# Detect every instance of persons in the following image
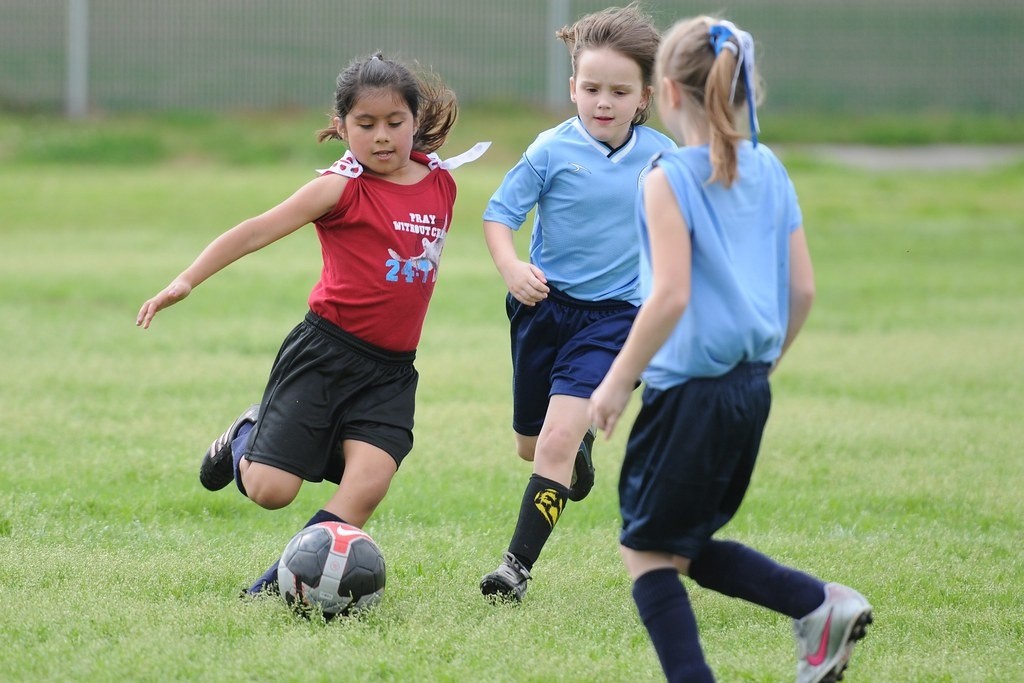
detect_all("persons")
[136,51,457,594]
[587,16,874,683]
[480,6,680,606]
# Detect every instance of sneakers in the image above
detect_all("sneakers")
[569,426,597,502]
[479,550,534,608]
[200,403,262,491]
[791,577,874,682]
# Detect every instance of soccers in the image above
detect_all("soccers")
[276,521,389,626]
